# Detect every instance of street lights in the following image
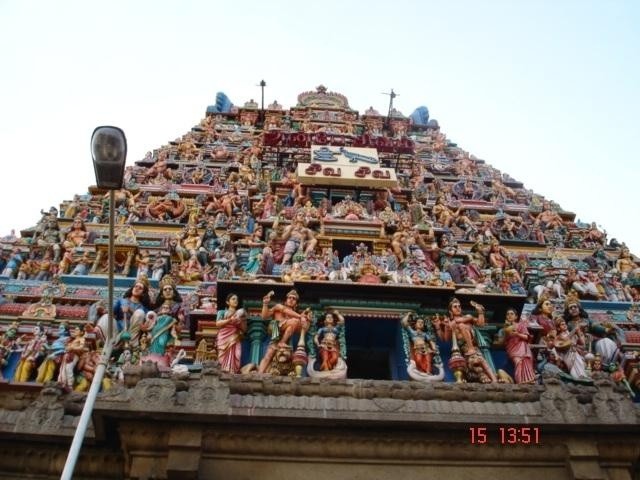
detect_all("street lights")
[395,142,404,174]
[58,127,126,480]
[275,139,282,167]
[255,80,266,130]
[384,89,396,129]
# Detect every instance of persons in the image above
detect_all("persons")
[2,110,640,393]
